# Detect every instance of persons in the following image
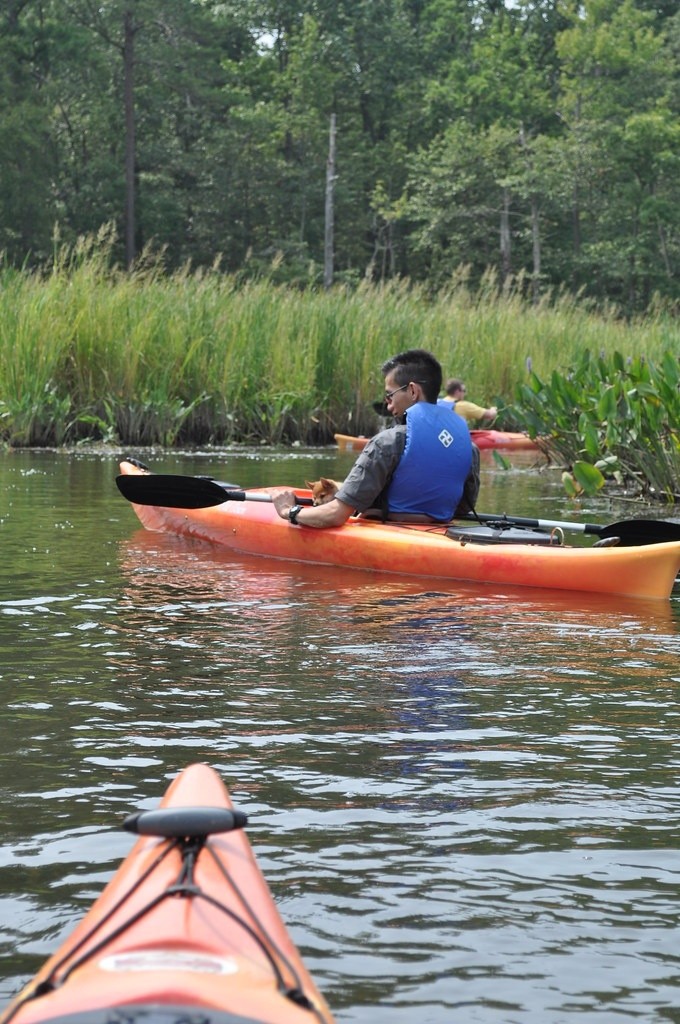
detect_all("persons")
[271,349,482,531]
[437,378,498,424]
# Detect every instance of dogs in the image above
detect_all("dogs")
[304,476,341,507]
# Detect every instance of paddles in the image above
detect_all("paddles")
[114,473,680,548]
[372,402,394,418]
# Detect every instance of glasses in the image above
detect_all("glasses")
[385,380,427,402]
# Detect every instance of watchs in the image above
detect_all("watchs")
[288,505,304,525]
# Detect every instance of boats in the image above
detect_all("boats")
[120,455,680,602]
[334,429,560,457]
